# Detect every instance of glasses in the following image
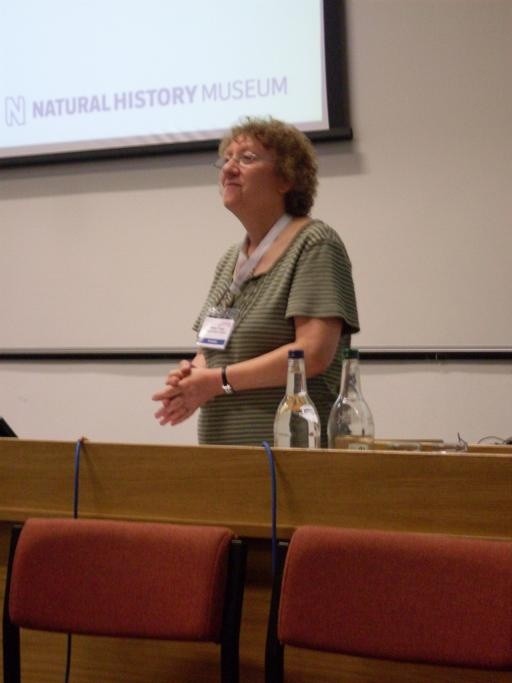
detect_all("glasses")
[215,149,278,168]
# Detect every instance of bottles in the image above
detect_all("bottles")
[272,347,323,448]
[324,348,378,448]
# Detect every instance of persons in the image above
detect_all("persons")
[151,113,361,449]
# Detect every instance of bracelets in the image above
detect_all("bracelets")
[221,363,239,395]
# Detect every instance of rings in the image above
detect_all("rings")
[183,406,189,412]
